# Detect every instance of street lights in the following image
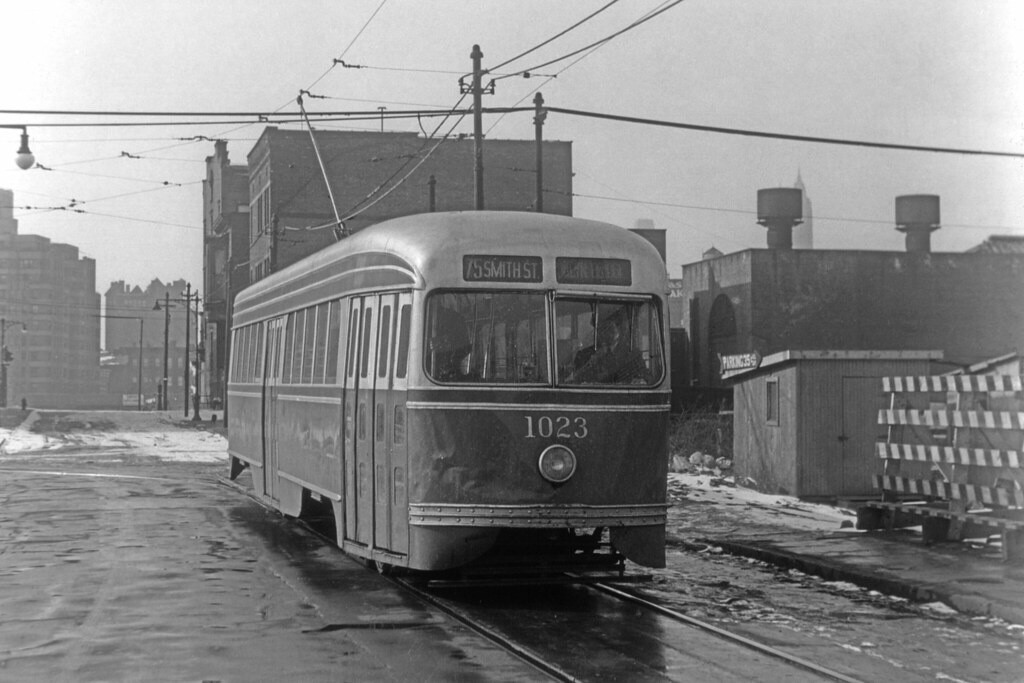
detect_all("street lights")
[0,319,28,410]
[152,298,201,421]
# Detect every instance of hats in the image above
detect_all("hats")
[590,308,624,326]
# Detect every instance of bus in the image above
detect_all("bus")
[227,209,673,591]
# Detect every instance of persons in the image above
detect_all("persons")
[575,307,650,385]
[428,307,471,380]
